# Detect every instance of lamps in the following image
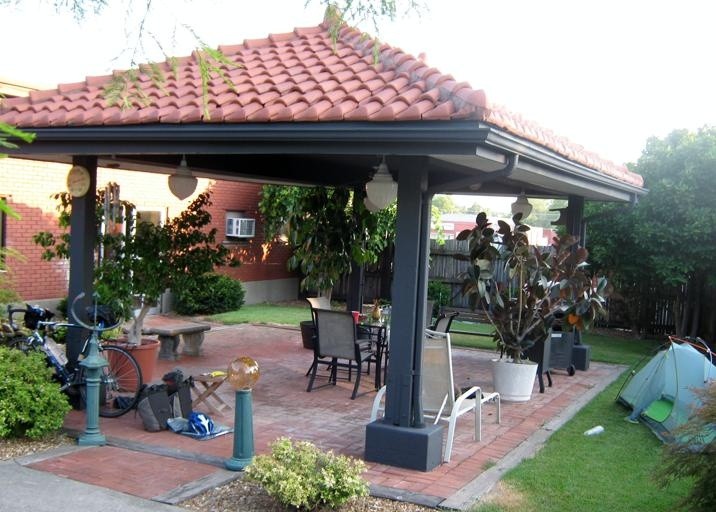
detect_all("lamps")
[167,154,198,198]
[365,154,400,211]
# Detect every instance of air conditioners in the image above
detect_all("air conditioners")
[227,218,257,240]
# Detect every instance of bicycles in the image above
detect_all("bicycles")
[7,298,143,421]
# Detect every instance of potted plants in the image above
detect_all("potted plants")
[452,210,614,402]
[257,185,447,351]
[30,185,162,386]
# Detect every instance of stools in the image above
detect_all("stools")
[174,371,230,416]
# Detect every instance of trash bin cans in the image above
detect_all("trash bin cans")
[300,321,315,349]
[548,318,576,376]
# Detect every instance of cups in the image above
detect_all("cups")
[353,311,359,324]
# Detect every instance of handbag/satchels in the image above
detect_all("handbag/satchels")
[137,391,173,431]
[170,385,193,418]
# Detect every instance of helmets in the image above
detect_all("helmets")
[188,412,214,439]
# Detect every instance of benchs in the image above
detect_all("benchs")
[142,321,207,359]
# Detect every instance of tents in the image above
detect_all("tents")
[613,333,716,459]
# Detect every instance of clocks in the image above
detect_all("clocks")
[66,167,90,200]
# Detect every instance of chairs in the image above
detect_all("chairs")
[281,297,501,464]
